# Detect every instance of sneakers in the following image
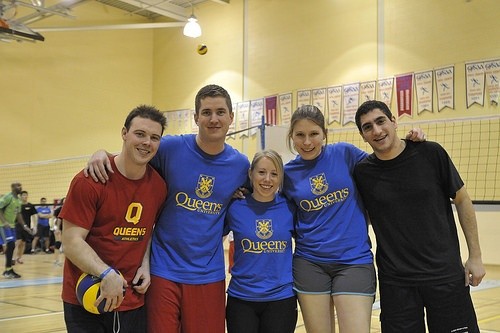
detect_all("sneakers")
[3,268,21,278]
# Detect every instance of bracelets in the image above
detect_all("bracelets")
[99,266,120,278]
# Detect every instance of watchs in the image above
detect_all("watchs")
[22,224,28,228]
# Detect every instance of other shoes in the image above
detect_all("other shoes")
[45,249,52,254]
[12,259,16,265]
[16,257,23,264]
[30,249,35,253]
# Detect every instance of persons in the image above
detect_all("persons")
[353,100,486,333]
[0,85,426,333]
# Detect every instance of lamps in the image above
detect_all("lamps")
[182,3,202,38]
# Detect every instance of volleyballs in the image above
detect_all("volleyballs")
[197,44,208,55]
[76,266,127,314]
